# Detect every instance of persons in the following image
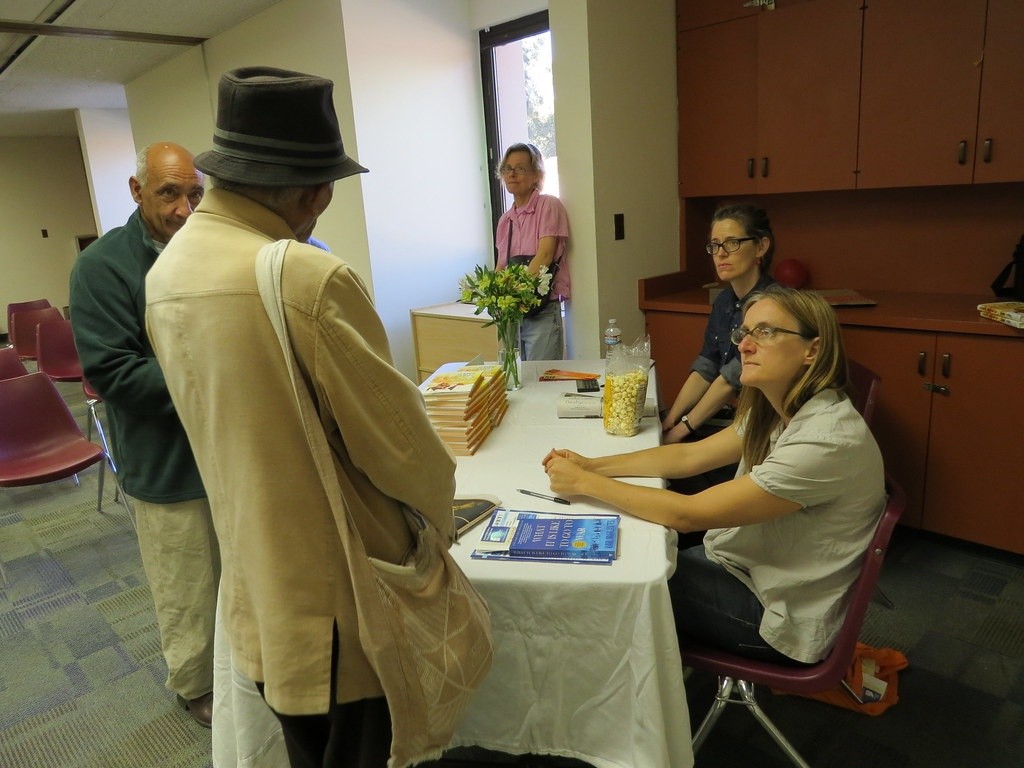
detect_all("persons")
[145,64,457,768]
[495,142,571,361]
[70,142,222,728]
[658,204,786,552]
[542,286,890,682]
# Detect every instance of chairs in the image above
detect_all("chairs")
[0,298,141,540]
[839,355,881,428]
[684,469,906,768]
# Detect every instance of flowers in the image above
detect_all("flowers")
[455,260,553,388]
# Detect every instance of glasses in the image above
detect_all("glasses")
[731,324,803,344]
[500,164,531,175]
[705,237,753,255]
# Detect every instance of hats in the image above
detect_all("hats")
[194,67,369,187]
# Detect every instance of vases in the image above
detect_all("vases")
[494,320,523,390]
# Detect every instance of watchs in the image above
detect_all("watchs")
[681,416,697,434]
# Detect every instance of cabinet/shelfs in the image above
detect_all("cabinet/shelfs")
[637,0,1024,556]
[410,300,503,384]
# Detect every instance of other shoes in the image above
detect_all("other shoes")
[176,692,212,729]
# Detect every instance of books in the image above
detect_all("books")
[415,365,510,458]
[555,390,657,419]
[540,368,601,381]
[467,508,620,565]
[976,300,1024,331]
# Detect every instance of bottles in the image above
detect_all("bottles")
[604,319,622,367]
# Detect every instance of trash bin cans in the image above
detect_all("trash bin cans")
[63,305,70,320]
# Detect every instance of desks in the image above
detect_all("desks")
[418,358,695,768]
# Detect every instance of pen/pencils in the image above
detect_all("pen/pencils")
[516,488,570,506]
[600,384,605,387]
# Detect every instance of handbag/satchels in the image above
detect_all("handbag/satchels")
[363,529,492,768]
